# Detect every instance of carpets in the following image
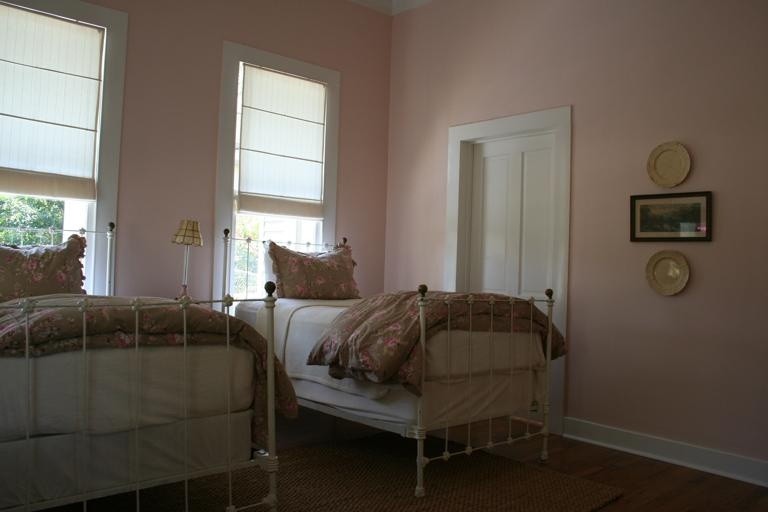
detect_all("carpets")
[29,430,626,511]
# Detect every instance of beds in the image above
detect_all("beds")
[0,221,278,511]
[222,228,554,499]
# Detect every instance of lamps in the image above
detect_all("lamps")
[170,219,204,302]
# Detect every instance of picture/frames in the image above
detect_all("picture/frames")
[629,191,713,243]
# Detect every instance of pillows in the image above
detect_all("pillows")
[267,237,362,300]
[0,233,87,304]
